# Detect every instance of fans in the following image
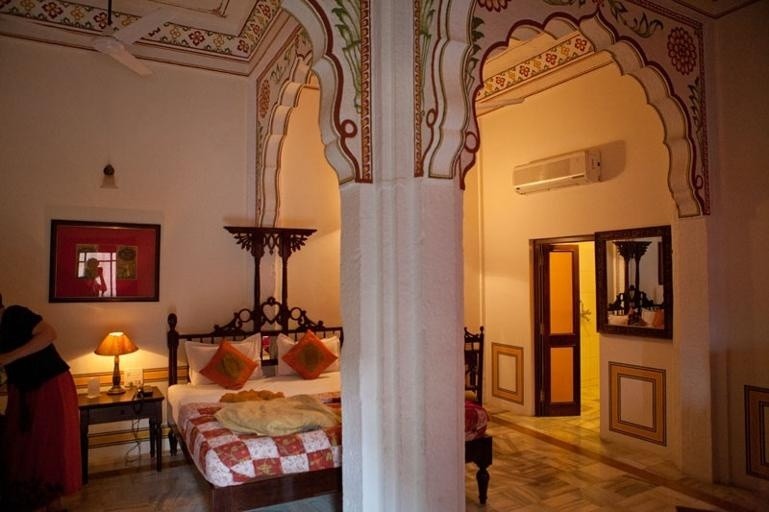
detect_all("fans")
[0,0,179,80]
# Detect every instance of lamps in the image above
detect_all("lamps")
[92,330,140,394]
[100,51,119,190]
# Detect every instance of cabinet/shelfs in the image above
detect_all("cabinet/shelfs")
[465,322,485,405]
[76,386,164,483]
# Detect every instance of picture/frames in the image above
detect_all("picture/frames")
[596,225,676,340]
[48,218,161,303]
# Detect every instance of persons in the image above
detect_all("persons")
[79,257,108,298]
[0,296,87,510]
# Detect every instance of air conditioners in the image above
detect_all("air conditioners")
[512,146,602,196]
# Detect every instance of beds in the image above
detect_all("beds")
[163,297,493,512]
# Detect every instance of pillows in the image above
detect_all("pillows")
[282,330,337,379]
[184,332,264,387]
[198,341,258,390]
[275,332,341,376]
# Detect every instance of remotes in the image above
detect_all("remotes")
[143,384,153,396]
